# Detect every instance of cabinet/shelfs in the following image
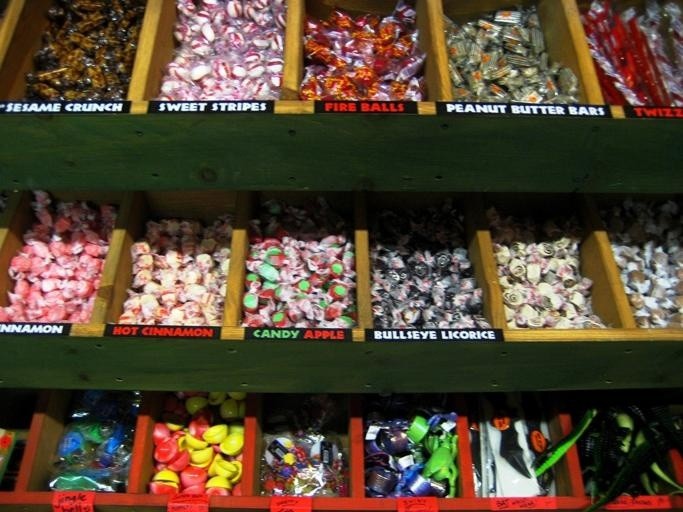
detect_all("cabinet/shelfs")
[0,188,682,341]
[0,0,683,116]
[0,384,682,511]
